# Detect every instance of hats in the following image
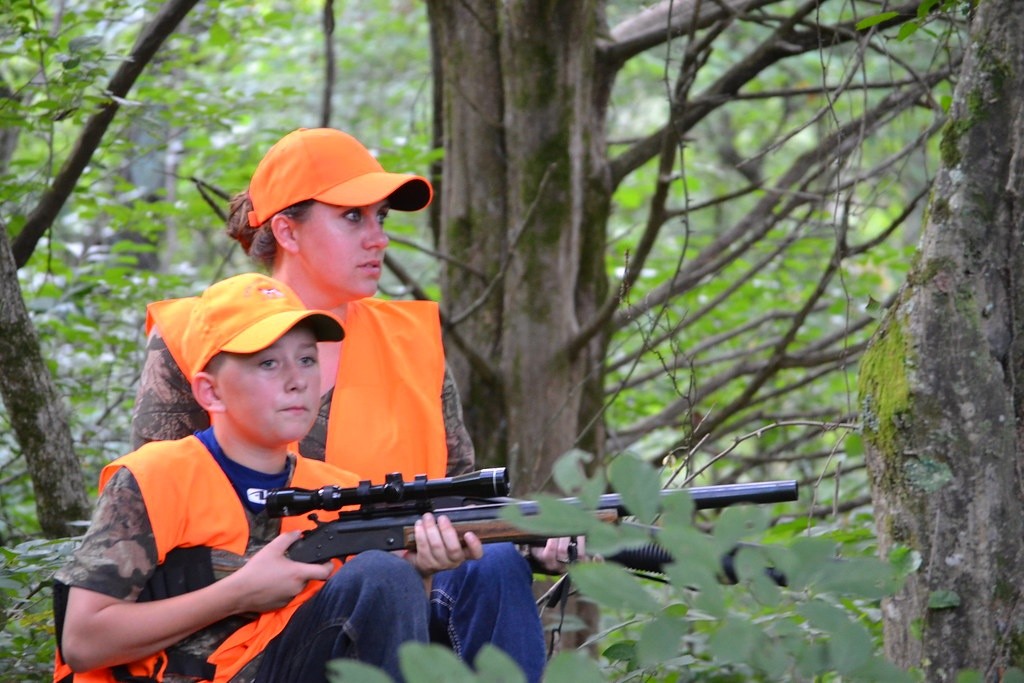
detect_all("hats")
[246,126,434,228]
[181,272,345,379]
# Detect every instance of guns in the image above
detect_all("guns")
[435,493,787,596]
[134,465,800,614]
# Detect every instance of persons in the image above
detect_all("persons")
[130,127,606,598]
[52,272,547,683]
